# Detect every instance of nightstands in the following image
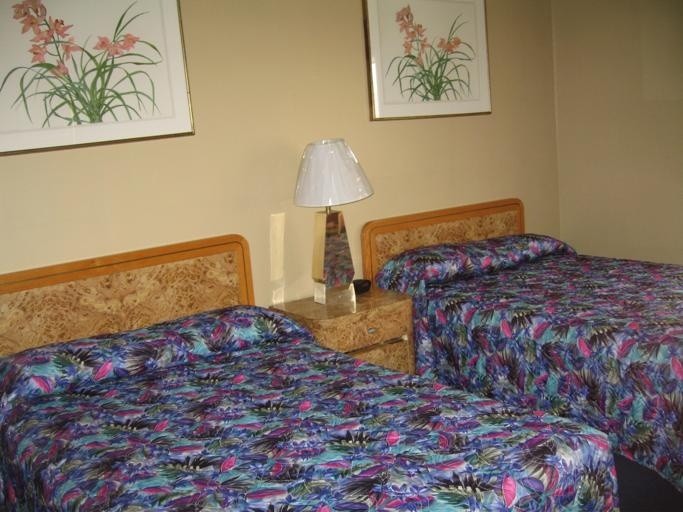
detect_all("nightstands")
[268,286,415,375]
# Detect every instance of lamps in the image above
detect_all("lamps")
[294,138,374,305]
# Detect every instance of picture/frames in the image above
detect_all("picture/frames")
[362,0,491,121]
[0,0,195,156]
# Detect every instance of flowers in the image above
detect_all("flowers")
[0,0,165,128]
[385,4,476,104]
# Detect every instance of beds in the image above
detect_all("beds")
[0,234,620,512]
[360,198,683,493]
